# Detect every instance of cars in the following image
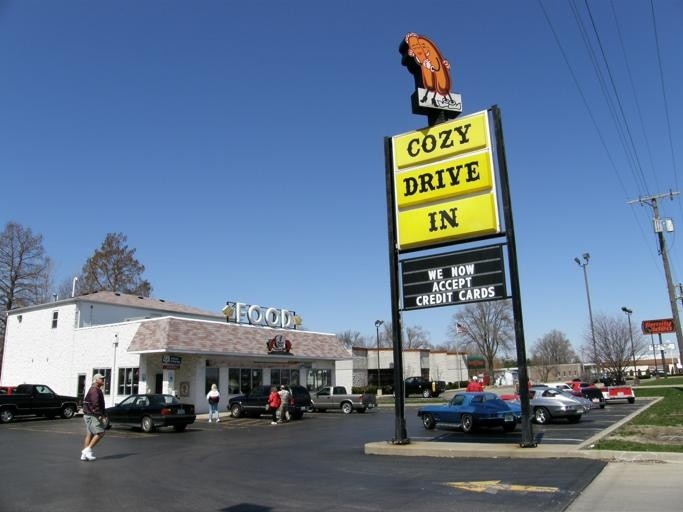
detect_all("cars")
[0,386,17,395]
[415,392,521,433]
[650,370,668,377]
[494,370,636,408]
[516,387,590,426]
[103,394,196,434]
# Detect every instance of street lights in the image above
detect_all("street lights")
[645,327,657,371]
[573,257,599,382]
[620,307,636,379]
[374,318,383,396]
[454,321,460,388]
[112,333,119,406]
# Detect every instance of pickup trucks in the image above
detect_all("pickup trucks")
[304,386,376,415]
[0,384,78,425]
[390,377,447,401]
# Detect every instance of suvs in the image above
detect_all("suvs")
[225,384,311,421]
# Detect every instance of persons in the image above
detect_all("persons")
[267,386,281,424]
[277,385,293,424]
[206,384,220,422]
[79,373,106,461]
[465,376,484,392]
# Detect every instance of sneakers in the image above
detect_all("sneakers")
[81,447,96,461]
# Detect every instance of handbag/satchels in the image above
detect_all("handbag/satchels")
[209,397,219,405]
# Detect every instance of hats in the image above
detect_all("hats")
[93,373,105,380]
